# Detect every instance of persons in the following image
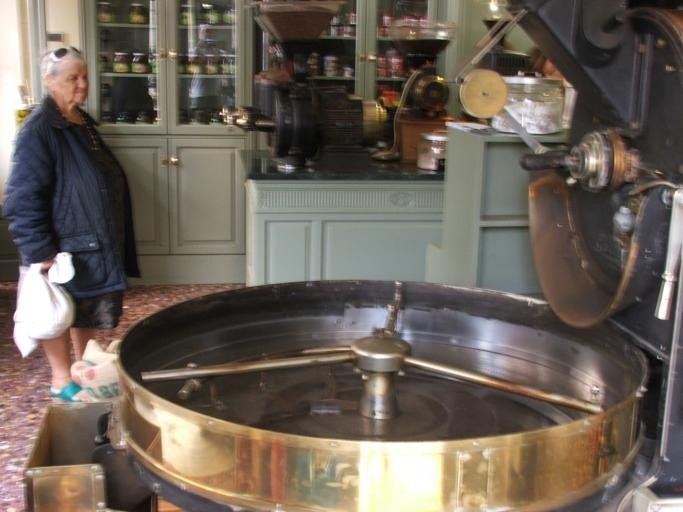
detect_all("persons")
[0,47,141,403]
[530,46,567,82]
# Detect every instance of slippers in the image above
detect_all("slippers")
[48,381,82,402]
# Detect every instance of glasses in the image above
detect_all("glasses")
[50,46,81,62]
